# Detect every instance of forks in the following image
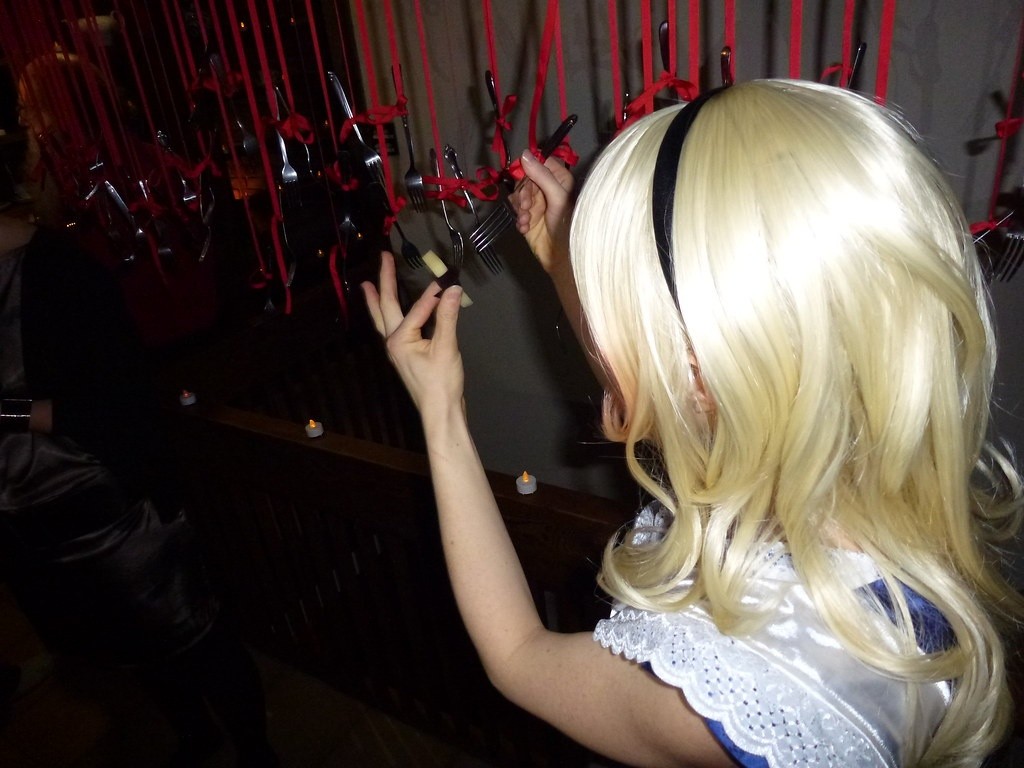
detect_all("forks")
[469,114,579,252]
[382,194,426,270]
[100,66,386,297]
[485,70,515,196]
[443,143,505,275]
[995,214,1024,284]
[429,147,464,270]
[391,63,427,214]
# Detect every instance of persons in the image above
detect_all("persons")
[0,51,282,767]
[361,77,1024,768]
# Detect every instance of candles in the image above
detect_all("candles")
[304,419,324,439]
[515,471,537,496]
[179,389,197,406]
